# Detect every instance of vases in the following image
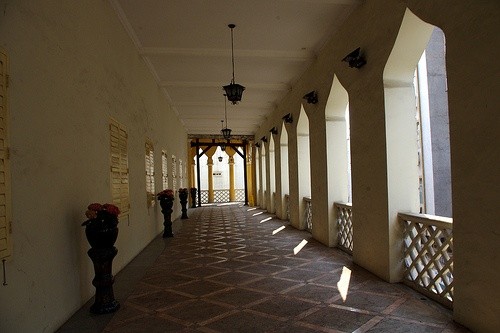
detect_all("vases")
[160,201,173,208]
[85,223,118,249]
[191,191,196,194]
[179,194,187,200]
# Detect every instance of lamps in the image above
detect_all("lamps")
[302,91,318,104]
[255,142,260,148]
[261,135,267,143]
[341,47,367,69]
[281,113,293,123]
[269,127,278,135]
[221,142,226,151]
[221,94,232,139]
[222,24,245,106]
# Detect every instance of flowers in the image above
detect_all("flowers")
[81,202,120,226]
[157,189,174,200]
[178,188,188,193]
[190,188,197,191]
